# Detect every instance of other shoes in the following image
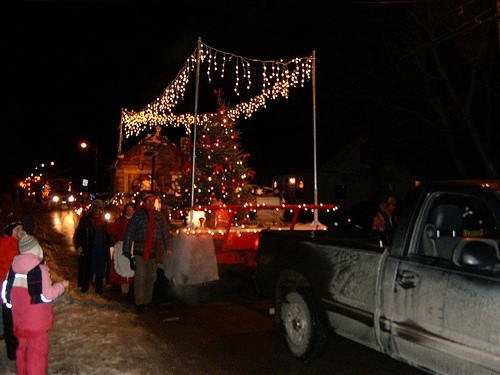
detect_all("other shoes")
[95,287,104,295]
[82,284,90,292]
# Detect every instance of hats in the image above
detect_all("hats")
[2,208,25,236]
[17,230,44,258]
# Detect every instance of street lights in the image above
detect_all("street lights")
[81,143,98,195]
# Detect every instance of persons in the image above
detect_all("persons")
[107,202,141,296]
[122,192,173,312]
[72,198,110,297]
[1,214,30,360]
[372,195,400,232]
[1,229,70,375]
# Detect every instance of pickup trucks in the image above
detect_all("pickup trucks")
[254,180,500,375]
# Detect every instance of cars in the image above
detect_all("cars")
[49,191,75,210]
